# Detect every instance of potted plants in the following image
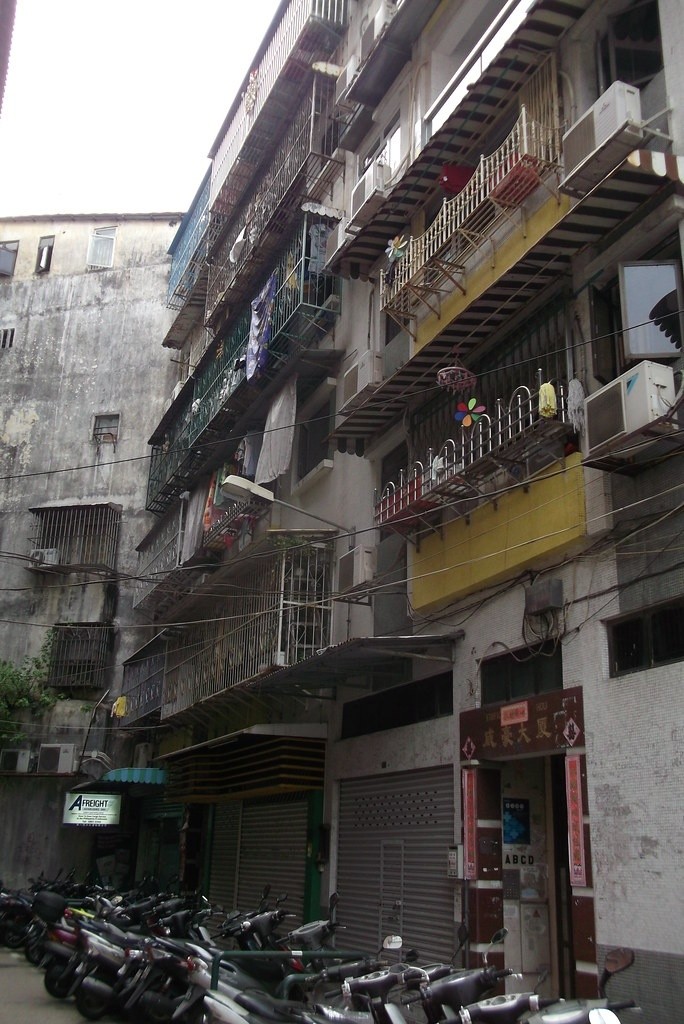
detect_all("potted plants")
[271,636,286,665]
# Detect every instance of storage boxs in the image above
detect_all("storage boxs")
[488,153,538,209]
[375,464,477,532]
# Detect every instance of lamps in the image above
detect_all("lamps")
[224,475,353,541]
[176,803,203,835]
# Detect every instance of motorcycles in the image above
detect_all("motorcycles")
[203,925,634,1024]
[0,867,346,1023]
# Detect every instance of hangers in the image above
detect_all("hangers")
[441,149,475,170]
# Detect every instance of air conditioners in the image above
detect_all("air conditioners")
[339,543,377,594]
[560,80,642,189]
[30,547,57,568]
[1,748,31,773]
[349,161,390,225]
[334,347,385,411]
[325,218,356,265]
[191,573,210,594]
[35,742,79,774]
[579,361,677,452]
[334,55,360,109]
[161,381,184,418]
[359,1,398,63]
[134,745,152,768]
